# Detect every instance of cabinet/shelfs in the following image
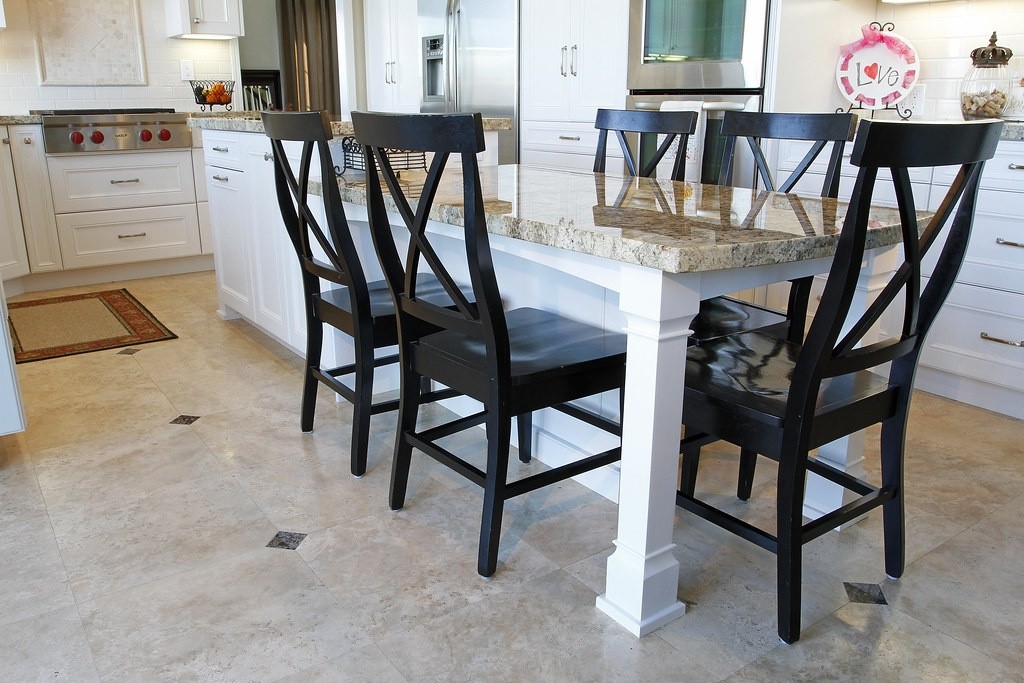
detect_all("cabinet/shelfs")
[203,127,360,375]
[361,0,420,114]
[767,130,1023,419]
[517,0,632,177]
[0,123,216,301]
[164,0,245,40]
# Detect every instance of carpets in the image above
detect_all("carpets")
[5,288,179,364]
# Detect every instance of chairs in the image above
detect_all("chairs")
[351,110,628,577]
[262,110,501,478]
[679,112,859,500]
[592,108,697,180]
[675,119,1005,647]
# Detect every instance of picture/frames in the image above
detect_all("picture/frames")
[241,69,283,110]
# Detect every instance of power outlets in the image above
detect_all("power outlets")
[180,59,195,80]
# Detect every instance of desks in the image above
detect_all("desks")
[296,164,940,639]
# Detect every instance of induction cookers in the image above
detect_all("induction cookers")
[30,107,194,154]
